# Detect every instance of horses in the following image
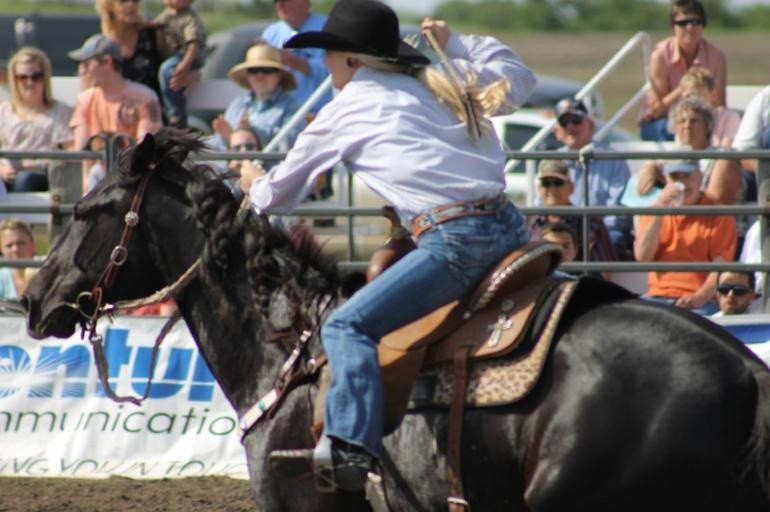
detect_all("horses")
[19,127,770,512]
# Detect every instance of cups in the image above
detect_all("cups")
[671,182,687,206]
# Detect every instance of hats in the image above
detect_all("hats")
[538,161,571,183]
[69,36,121,63]
[555,98,586,120]
[664,145,697,174]
[283,0,431,66]
[229,46,297,91]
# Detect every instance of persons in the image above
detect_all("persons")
[0,1,770,317]
[239,2,536,493]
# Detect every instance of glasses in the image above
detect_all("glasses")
[542,179,563,187]
[15,71,43,81]
[247,67,278,75]
[558,115,582,125]
[676,19,699,26]
[718,285,750,297]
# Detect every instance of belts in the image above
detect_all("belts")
[411,192,510,238]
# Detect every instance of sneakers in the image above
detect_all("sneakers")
[267,447,369,491]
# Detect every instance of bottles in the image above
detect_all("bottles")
[692,59,702,67]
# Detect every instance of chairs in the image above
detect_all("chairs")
[291,160,401,264]
